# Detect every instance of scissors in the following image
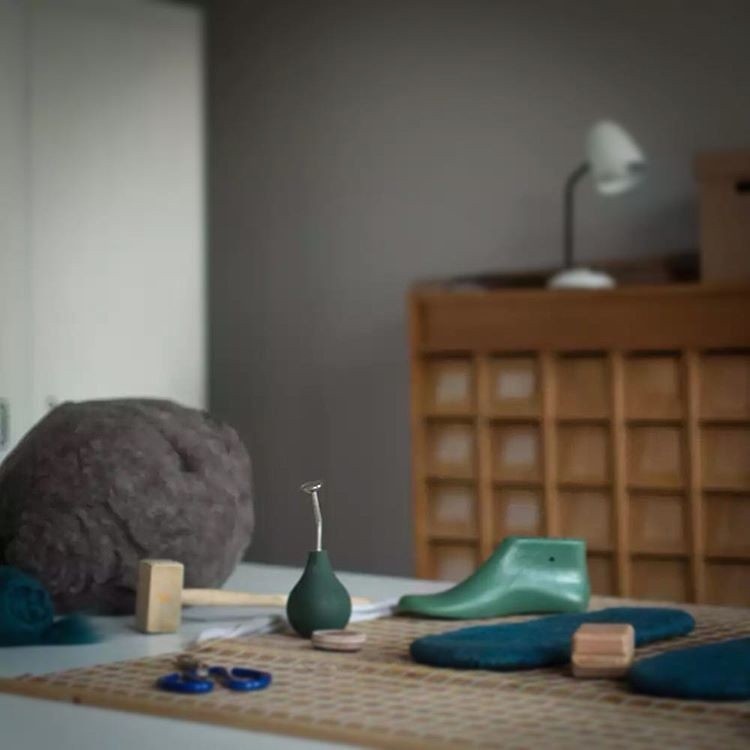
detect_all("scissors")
[155,651,274,695]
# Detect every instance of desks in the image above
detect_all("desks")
[0,564,750,748]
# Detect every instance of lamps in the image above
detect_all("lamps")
[548,122,651,291]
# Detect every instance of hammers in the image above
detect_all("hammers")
[134,556,368,635]
